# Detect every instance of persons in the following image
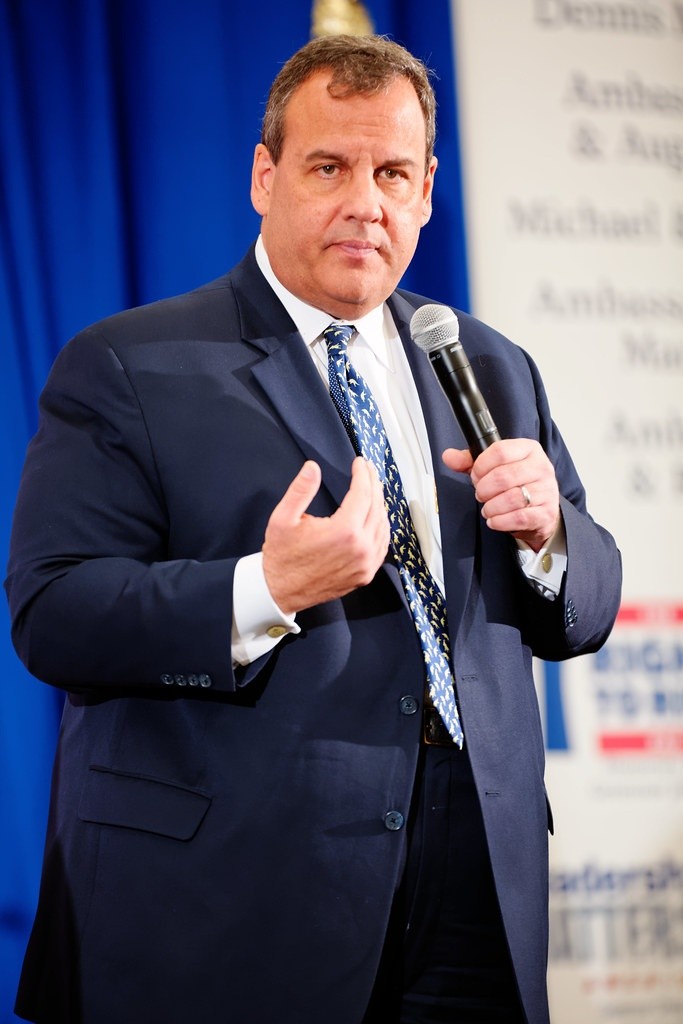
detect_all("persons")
[2,34,622,1024]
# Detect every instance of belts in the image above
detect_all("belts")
[420,708,452,746]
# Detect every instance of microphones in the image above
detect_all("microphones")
[410,305,501,465]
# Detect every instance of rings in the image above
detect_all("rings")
[519,485,531,508]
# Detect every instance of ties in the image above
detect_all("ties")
[322,324,464,751]
